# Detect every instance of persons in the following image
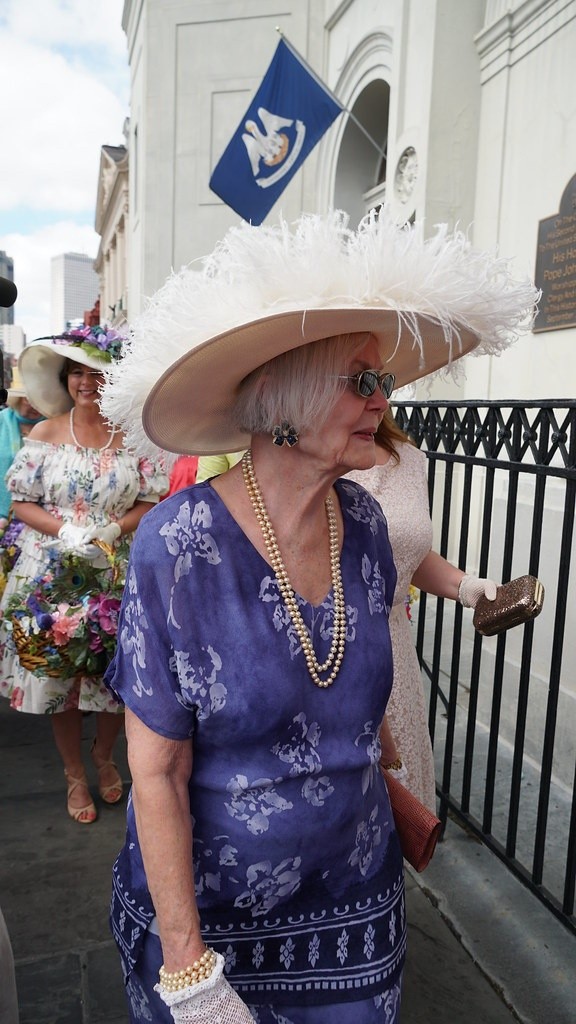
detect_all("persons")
[95,215,542,1024]
[338,404,502,814]
[0,325,251,822]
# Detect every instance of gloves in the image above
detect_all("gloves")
[71,522,122,560]
[459,572,504,608]
[153,947,257,1024]
[384,760,408,789]
[56,520,88,557]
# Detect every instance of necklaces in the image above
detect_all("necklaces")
[243,452,346,687]
[70,407,115,450]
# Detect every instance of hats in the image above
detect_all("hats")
[101,214,539,463]
[18,323,132,419]
[6,384,28,408]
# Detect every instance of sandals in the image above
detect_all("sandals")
[64,768,96,824]
[93,751,123,804]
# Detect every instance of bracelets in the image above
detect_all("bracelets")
[382,757,403,771]
[158,950,217,993]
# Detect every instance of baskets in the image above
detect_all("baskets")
[12,536,125,682]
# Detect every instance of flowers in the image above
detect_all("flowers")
[3,548,122,681]
[48,325,124,359]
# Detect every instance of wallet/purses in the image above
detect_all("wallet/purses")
[379,763,442,873]
[472,575,545,637]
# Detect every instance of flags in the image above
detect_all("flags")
[209,38,342,226]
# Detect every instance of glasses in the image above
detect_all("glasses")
[334,369,395,400]
